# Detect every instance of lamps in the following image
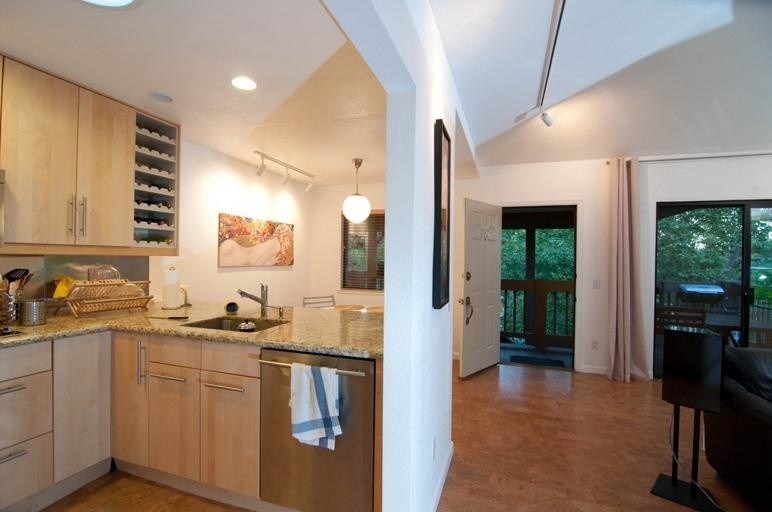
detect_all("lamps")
[538,105,556,128]
[340,159,372,225]
[252,150,317,193]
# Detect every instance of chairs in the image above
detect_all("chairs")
[302,295,337,309]
[703,346,772,511]
[655,286,771,348]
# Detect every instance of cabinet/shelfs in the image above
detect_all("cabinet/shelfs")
[199,341,261,506]
[112,331,148,469]
[136,107,181,257]
[0,340,55,510]
[144,335,199,482]
[1,54,136,256]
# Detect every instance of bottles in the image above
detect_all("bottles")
[149,183,172,191]
[136,216,171,226]
[136,199,172,209]
[135,234,172,244]
[162,266,181,309]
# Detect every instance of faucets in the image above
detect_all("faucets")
[235,281,269,319]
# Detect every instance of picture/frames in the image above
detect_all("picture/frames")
[433,119,451,309]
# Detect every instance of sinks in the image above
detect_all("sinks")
[178,314,293,334]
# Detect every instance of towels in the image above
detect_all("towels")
[288,362,342,452]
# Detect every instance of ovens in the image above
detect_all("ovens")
[257,347,373,512]
